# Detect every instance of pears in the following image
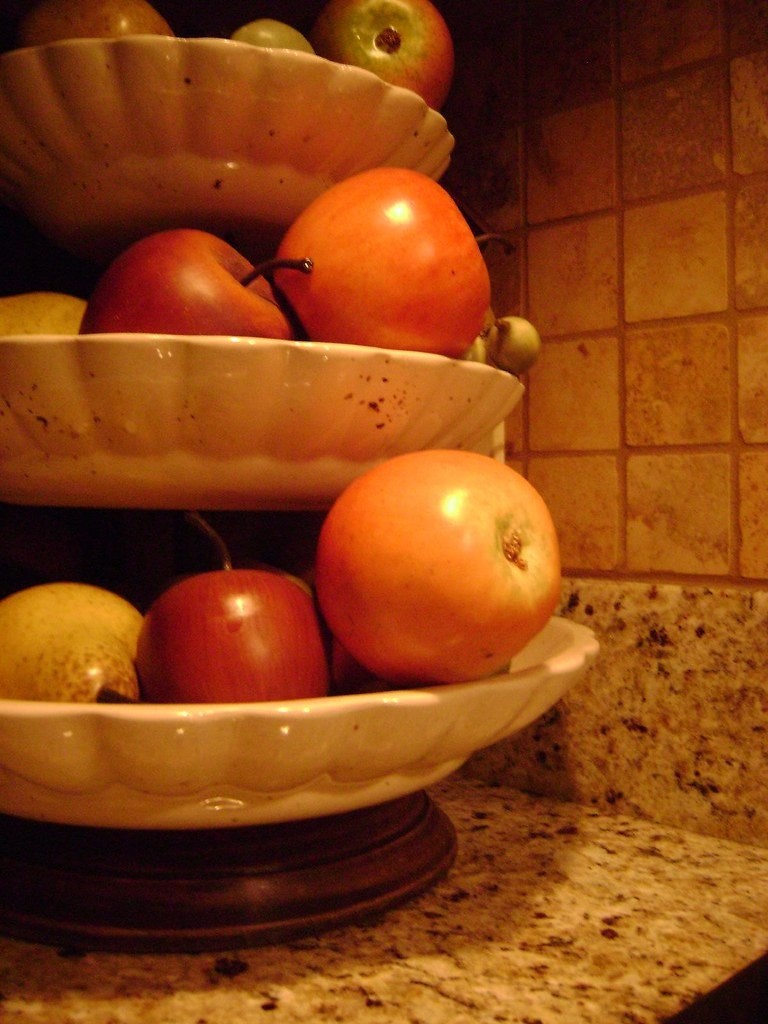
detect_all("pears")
[0,580,147,703]
[0,293,88,336]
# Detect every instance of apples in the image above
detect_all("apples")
[186,0,453,110]
[78,166,492,358]
[20,0,175,45]
[141,448,563,703]
